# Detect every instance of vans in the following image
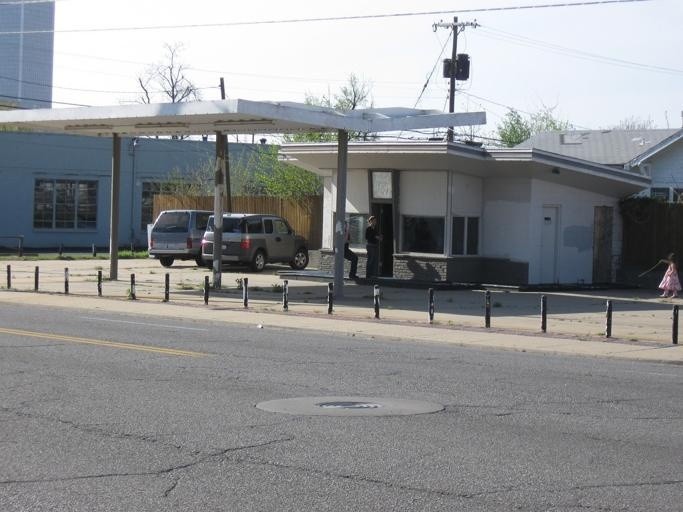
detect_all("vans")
[200,213,308,272]
[148,209,235,266]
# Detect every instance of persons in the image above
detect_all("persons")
[343,220,360,279]
[365,215,384,280]
[658,252,683,298]
